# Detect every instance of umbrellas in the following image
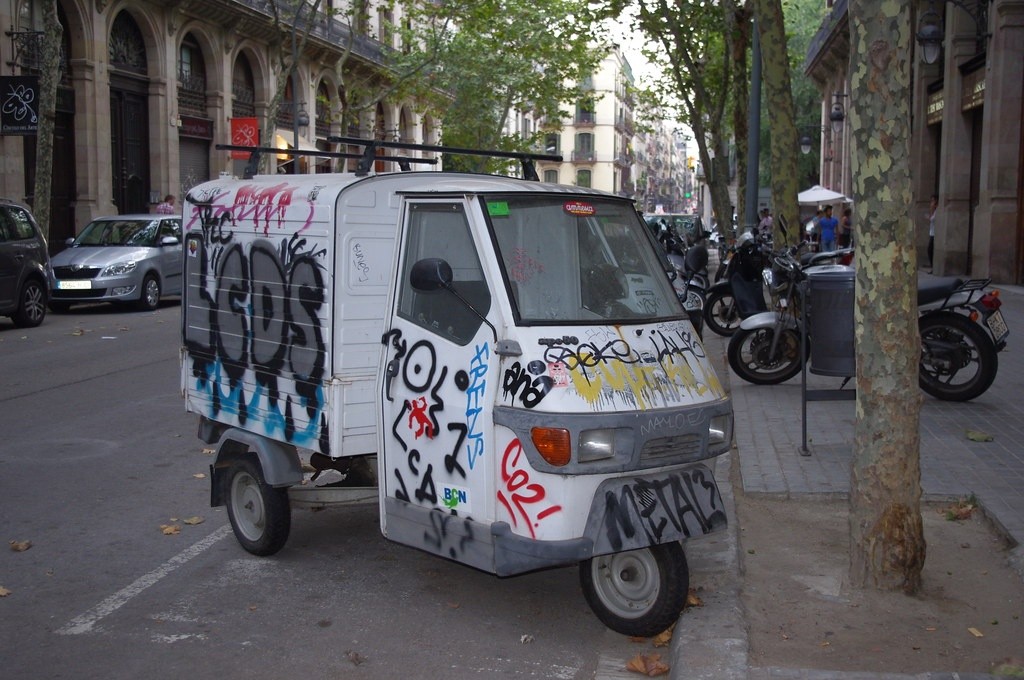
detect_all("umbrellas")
[843,198,853,204]
[797,185,846,211]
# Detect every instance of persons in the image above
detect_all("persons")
[811,210,823,253]
[925,195,938,274]
[156,195,176,214]
[841,208,854,248]
[758,208,773,240]
[817,204,839,252]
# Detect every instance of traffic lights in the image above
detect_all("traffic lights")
[685,193,691,204]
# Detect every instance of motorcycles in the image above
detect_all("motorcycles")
[702,216,1010,401]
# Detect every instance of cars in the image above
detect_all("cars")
[41,213,183,312]
[0,196,53,328]
[587,212,710,336]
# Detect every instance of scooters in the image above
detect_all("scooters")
[179,137,734,637]
